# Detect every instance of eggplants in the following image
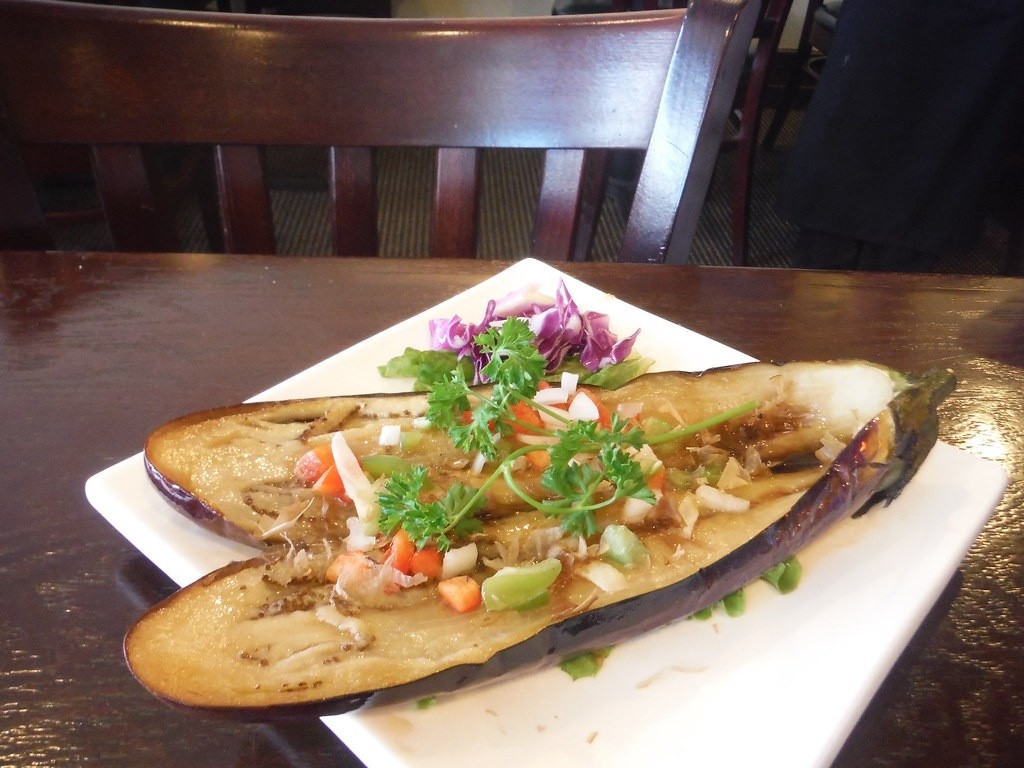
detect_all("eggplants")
[122,356,961,725]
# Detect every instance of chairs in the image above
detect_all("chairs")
[553,0,794,261]
[2,0,771,247]
[765,0,843,150]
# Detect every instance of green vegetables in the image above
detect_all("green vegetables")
[372,317,761,557]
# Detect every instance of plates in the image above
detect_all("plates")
[82,255,1013,768]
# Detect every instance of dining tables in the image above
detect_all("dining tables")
[0,243,1024,767]
